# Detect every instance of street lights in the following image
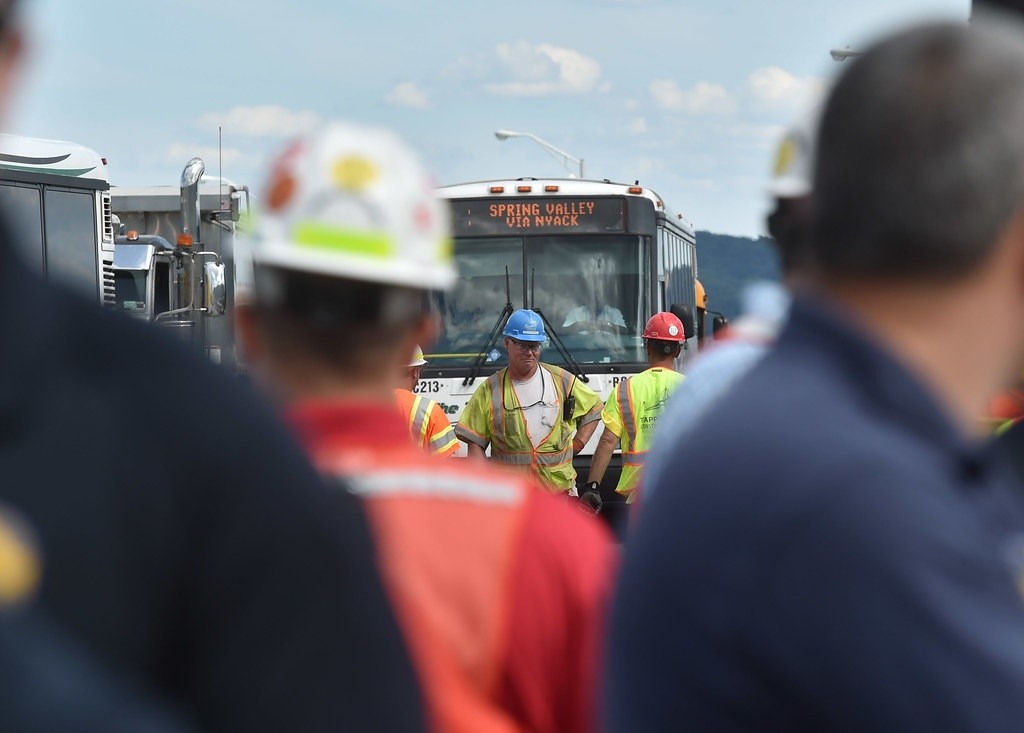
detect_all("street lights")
[493,130,585,179]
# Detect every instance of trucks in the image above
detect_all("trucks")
[0,132,256,368]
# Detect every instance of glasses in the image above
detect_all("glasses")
[510,338,541,352]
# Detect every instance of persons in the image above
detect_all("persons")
[452,309,604,497]
[0,1,1024,733]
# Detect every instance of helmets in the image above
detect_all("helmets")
[502,309,547,342]
[406,342,428,368]
[641,311,686,342]
[246,121,460,294]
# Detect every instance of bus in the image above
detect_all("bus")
[411,176,730,543]
